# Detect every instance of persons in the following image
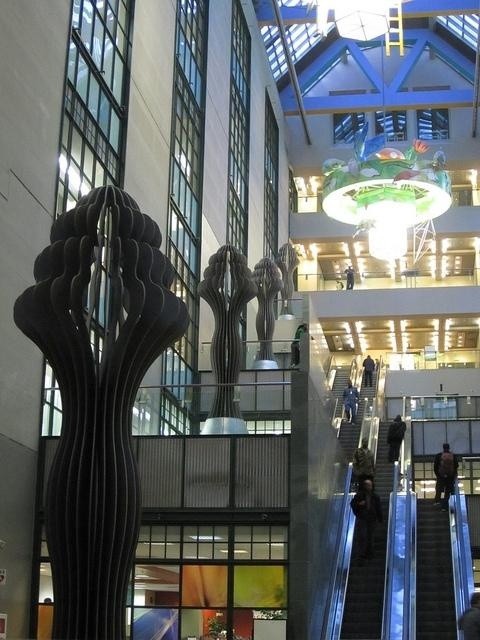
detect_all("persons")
[351,480,383,561]
[291,322,314,368]
[362,355,375,387]
[353,438,377,484]
[342,380,360,425]
[458,593,480,639]
[386,414,406,462]
[433,442,458,503]
[335,278,344,289]
[345,265,356,288]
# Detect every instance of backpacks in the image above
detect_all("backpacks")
[437,453,455,479]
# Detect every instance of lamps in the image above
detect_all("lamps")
[327,0,394,43]
[313,38,455,266]
